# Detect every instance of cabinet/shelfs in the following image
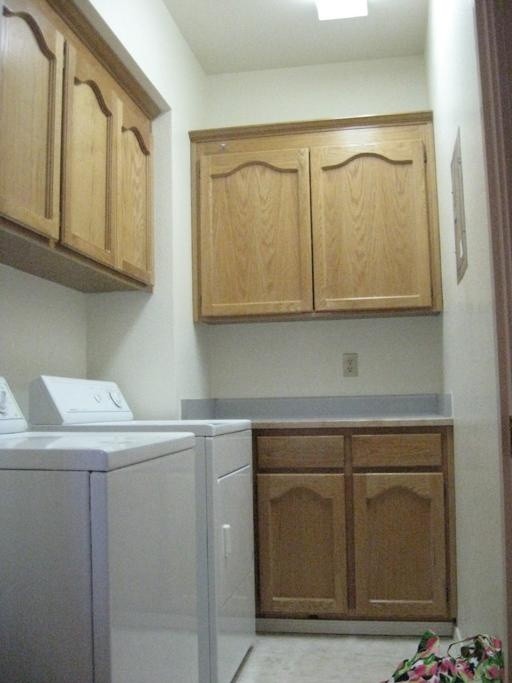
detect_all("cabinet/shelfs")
[48,1,154,292]
[252,426,457,637]
[188,110,442,325]
[3,1,66,276]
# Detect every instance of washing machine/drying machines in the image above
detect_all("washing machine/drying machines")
[1,378,200,682]
[28,376,256,681]
